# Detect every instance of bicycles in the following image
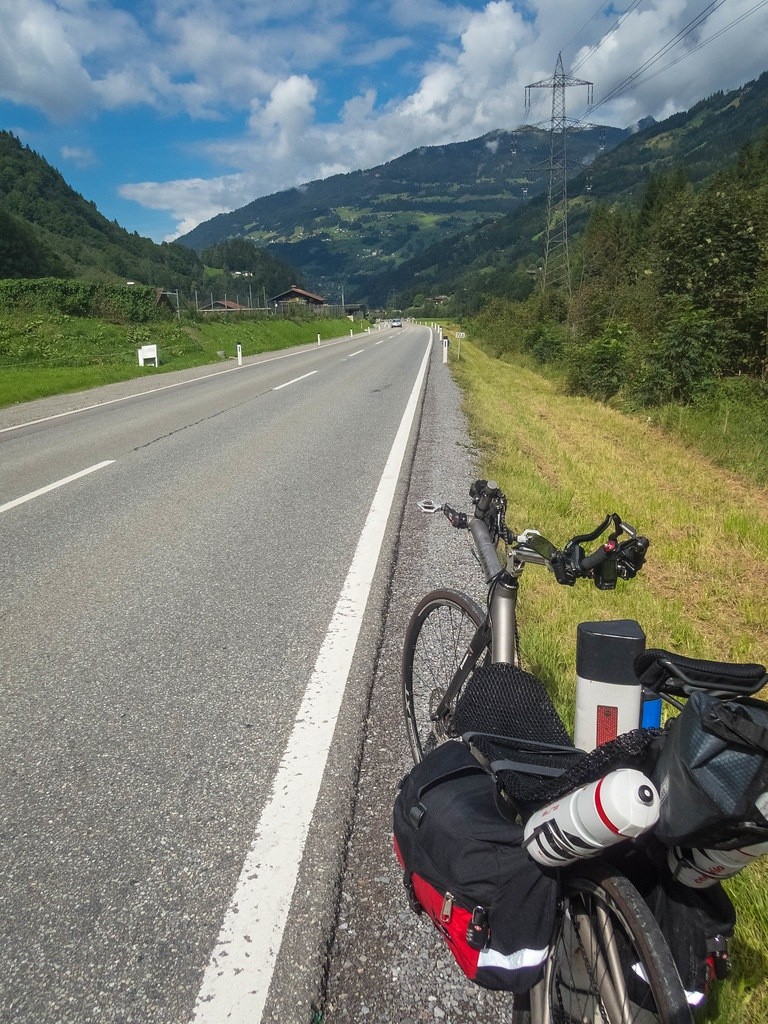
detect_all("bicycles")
[397,479,694,1022]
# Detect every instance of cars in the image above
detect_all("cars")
[391,319,402,328]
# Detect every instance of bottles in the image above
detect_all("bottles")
[524,769,660,865]
[668,789,768,888]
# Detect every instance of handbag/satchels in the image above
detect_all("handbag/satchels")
[391,739,563,994]
[610,863,736,1024]
[650,696,768,849]
